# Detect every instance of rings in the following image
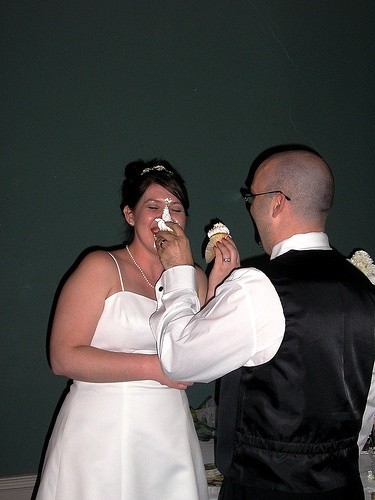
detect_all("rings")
[160,239,167,249]
[223,258,231,262]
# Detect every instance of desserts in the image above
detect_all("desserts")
[154,207,174,232]
[207,222,230,246]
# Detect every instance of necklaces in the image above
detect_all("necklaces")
[126,245,155,289]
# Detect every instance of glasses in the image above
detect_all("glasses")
[244,187,290,207]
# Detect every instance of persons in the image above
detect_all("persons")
[149,150,375,500]
[35,158,241,500]
